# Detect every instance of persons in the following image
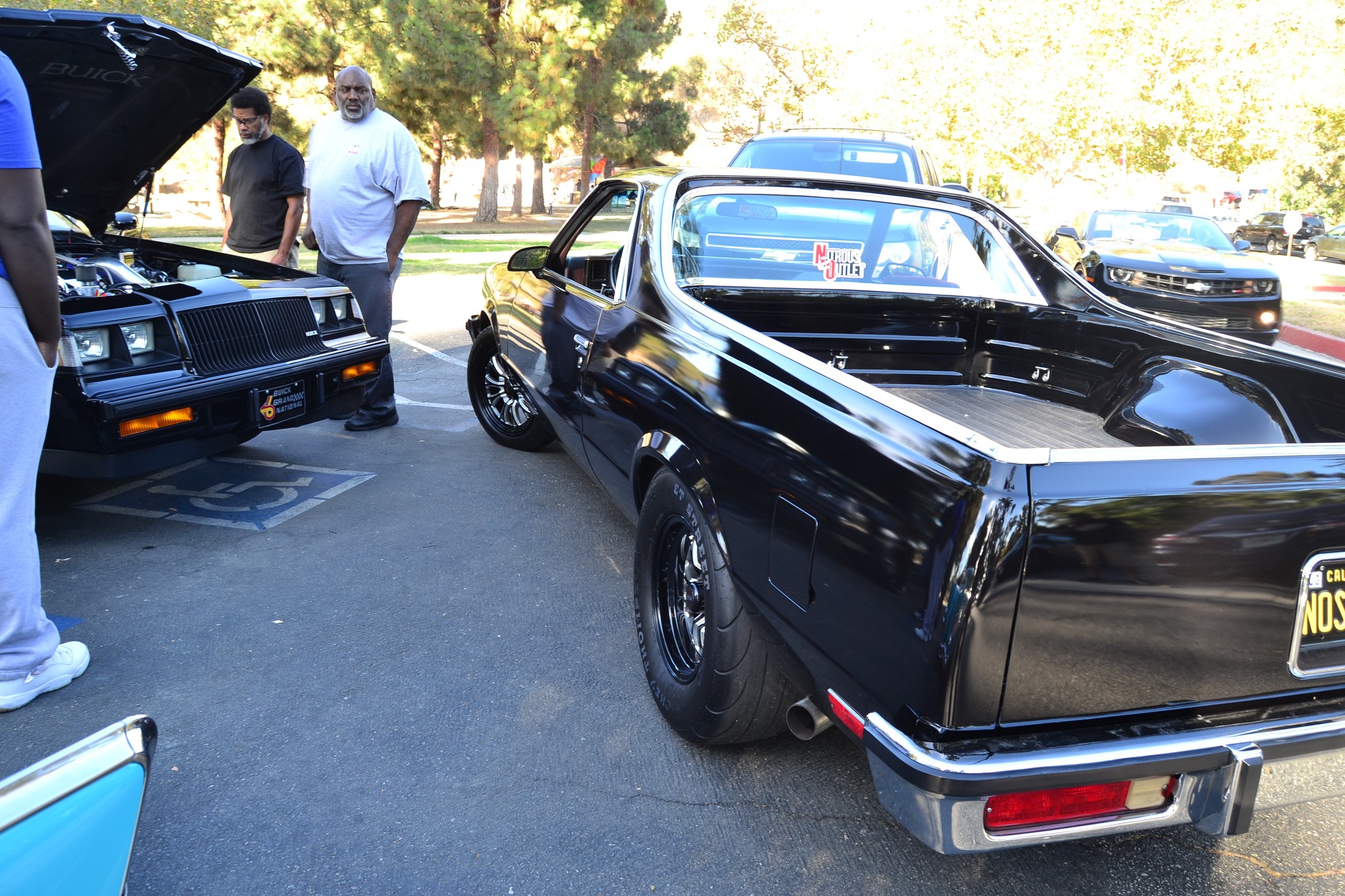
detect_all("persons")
[219,87,307,269]
[628,190,638,200]
[589,185,594,192]
[567,177,581,205]
[0,45,94,711]
[302,66,433,433]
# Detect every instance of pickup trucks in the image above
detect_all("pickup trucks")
[466,166,1345,862]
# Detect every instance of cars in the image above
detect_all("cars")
[1213,188,1272,210]
[1231,209,1327,257]
[682,135,972,280]
[1055,210,1284,346]
[1149,196,1196,234]
[1,8,399,520]
[1303,225,1345,262]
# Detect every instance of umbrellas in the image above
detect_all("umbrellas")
[549,156,671,207]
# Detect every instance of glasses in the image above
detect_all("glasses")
[231,115,262,125]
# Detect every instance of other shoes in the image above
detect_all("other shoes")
[329,409,358,420]
[344,406,400,431]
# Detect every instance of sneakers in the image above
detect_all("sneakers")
[0,641,91,713]
